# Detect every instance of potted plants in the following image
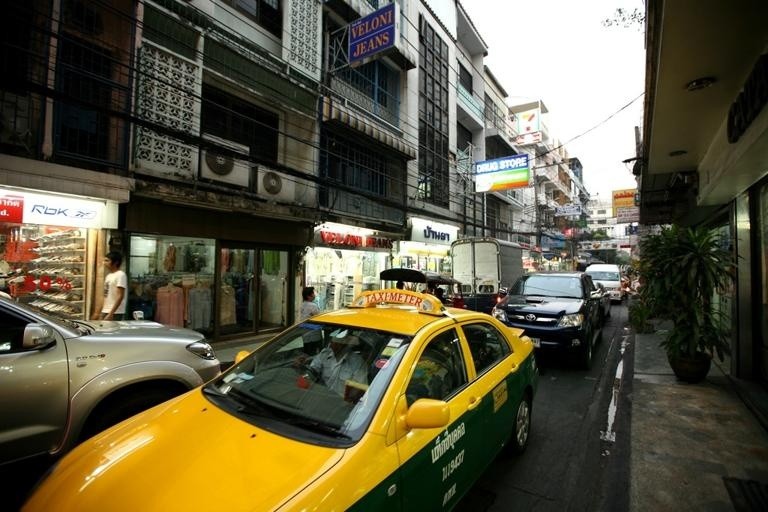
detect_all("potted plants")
[625,219,747,385]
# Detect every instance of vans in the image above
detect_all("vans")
[585,264,625,300]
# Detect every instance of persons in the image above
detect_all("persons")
[0,244,22,290]
[434,287,448,304]
[299,285,320,320]
[89,251,128,322]
[308,331,370,401]
[396,279,404,289]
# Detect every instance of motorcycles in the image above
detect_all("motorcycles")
[453,285,467,308]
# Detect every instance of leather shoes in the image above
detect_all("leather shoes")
[28,230,84,314]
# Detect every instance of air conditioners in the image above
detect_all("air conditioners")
[199,130,297,202]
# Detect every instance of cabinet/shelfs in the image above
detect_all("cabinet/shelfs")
[26,229,86,318]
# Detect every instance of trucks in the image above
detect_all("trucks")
[450,237,523,298]
